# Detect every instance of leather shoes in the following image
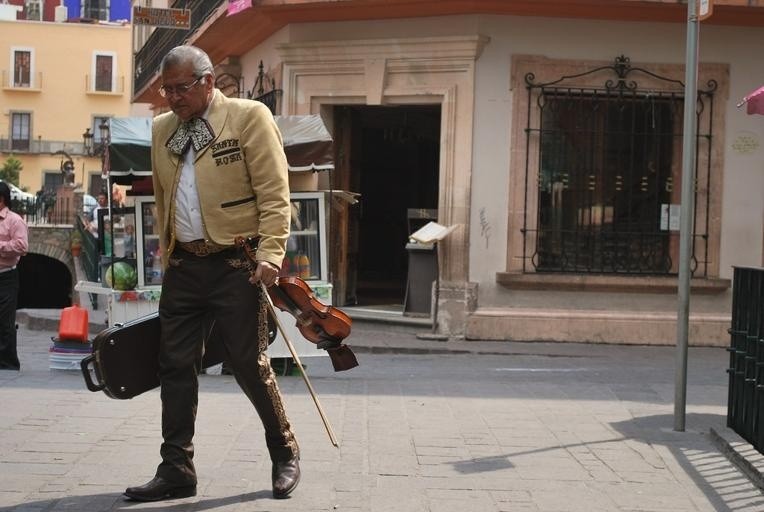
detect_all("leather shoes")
[123,469,198,502]
[271,447,300,500]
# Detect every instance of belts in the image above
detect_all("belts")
[175,239,230,256]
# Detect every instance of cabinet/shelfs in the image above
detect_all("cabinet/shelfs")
[134,192,328,290]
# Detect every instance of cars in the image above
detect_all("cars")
[81,195,103,226]
[0,178,38,214]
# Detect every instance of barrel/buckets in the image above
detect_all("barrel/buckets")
[59,303,89,343]
[55,6,68,22]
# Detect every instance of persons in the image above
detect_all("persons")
[83,185,134,260]
[0,180,29,370]
[122,44,301,499]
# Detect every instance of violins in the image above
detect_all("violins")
[235,235,351,344]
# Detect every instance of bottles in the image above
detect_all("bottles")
[144,208,154,234]
[144,251,161,284]
[293,252,311,279]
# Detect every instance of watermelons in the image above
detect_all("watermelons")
[105,261,137,290]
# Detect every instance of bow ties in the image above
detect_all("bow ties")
[164,118,216,155]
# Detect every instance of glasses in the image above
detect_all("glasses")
[158,75,203,98]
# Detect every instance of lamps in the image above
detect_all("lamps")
[81,128,95,157]
